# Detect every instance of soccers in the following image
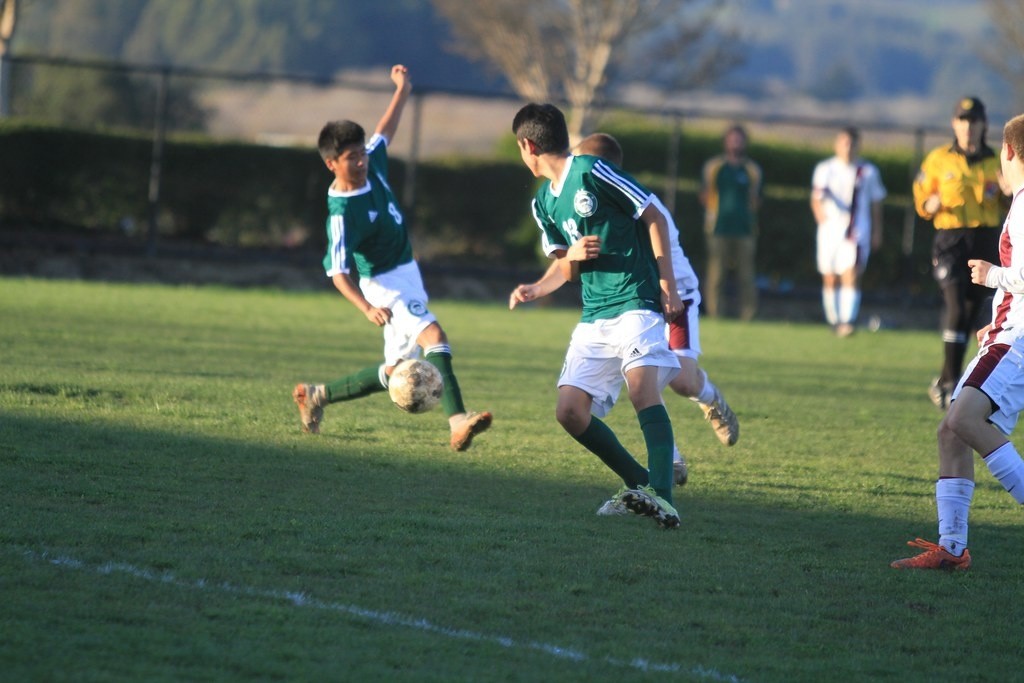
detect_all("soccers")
[388,357,445,414]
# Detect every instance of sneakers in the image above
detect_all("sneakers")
[891,538,971,570]
[597,486,632,515]
[672,461,686,484]
[293,383,324,435]
[701,385,738,446]
[451,411,494,451]
[622,483,681,530]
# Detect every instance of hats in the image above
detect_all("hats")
[955,97,983,118]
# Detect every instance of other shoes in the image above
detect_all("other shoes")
[835,322,856,338]
[929,379,952,407]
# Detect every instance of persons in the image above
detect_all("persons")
[912,96,1011,409]
[891,113,1024,568]
[701,126,763,322]
[810,126,888,337]
[509,133,739,487]
[293,64,493,451]
[512,103,685,528]
